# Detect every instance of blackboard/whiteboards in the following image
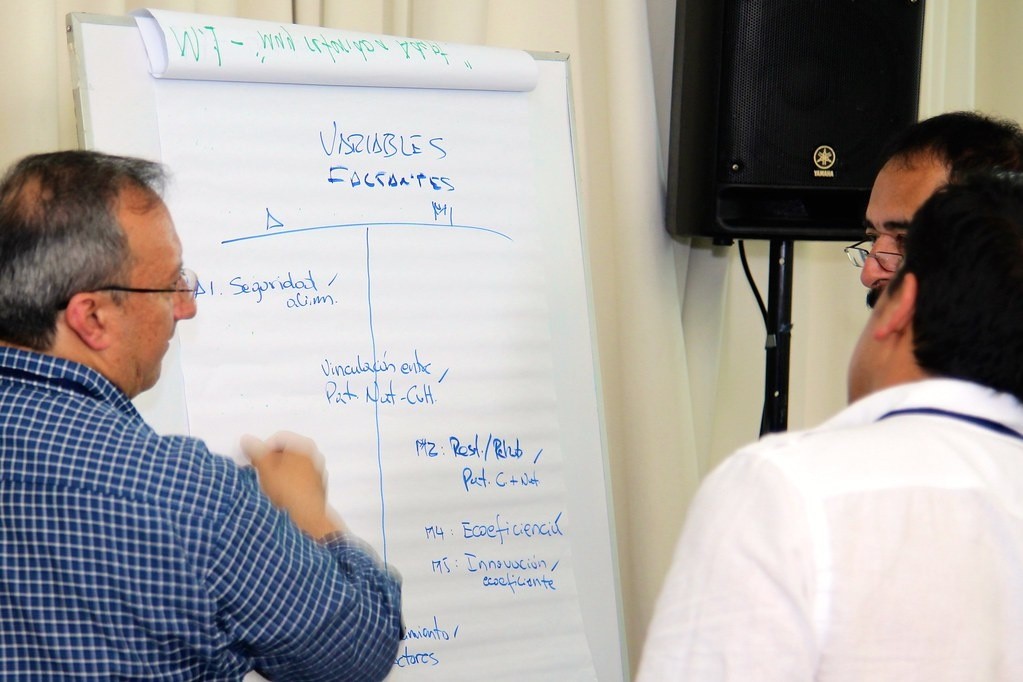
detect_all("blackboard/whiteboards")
[65,11,632,682]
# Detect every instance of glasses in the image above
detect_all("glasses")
[843,236,905,273]
[866,278,894,308]
[56,270,199,310]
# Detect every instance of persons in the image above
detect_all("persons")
[846,110,1023,307]
[632,173,1023,682]
[0,150,404,682]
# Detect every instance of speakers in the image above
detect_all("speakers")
[667,0,927,246]
